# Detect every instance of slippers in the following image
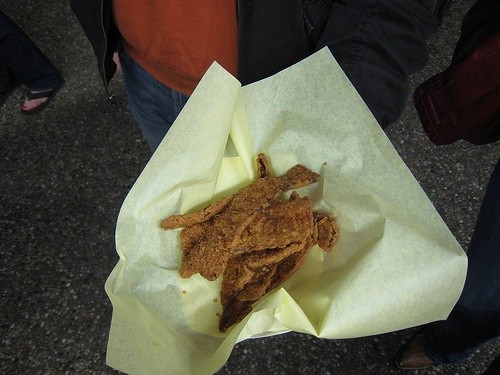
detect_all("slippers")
[21,79,65,115]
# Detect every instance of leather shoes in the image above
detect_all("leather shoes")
[394,332,435,369]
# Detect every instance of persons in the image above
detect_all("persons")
[0,11,65,116]
[396,0,500,369]
[71,0,451,154]
